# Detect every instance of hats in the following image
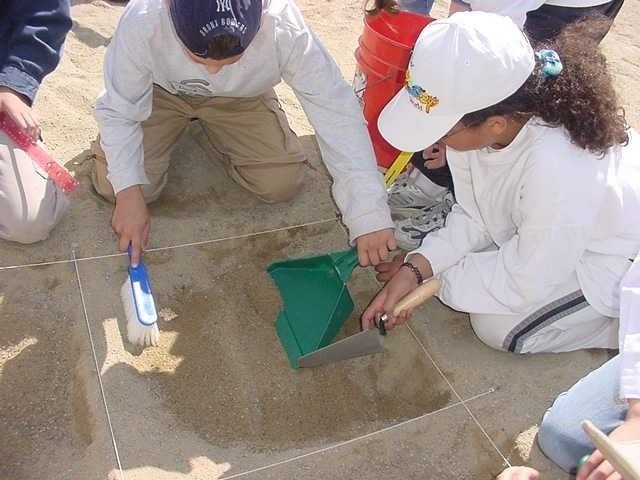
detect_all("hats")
[377,12,535,154]
[169,0,262,61]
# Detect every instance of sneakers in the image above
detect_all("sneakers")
[393,191,456,251]
[380,172,448,214]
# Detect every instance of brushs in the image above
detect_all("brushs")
[120,243,160,347]
[0,112,79,192]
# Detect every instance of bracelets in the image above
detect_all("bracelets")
[401,260,425,287]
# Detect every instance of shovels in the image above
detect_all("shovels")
[298,279,440,368]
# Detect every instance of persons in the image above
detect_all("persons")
[381,1,625,254]
[491,253,640,480]
[359,10,640,353]
[1,0,76,245]
[91,1,399,266]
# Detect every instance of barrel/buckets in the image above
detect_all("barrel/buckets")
[359,9,438,71]
[354,47,406,170]
[357,34,408,85]
